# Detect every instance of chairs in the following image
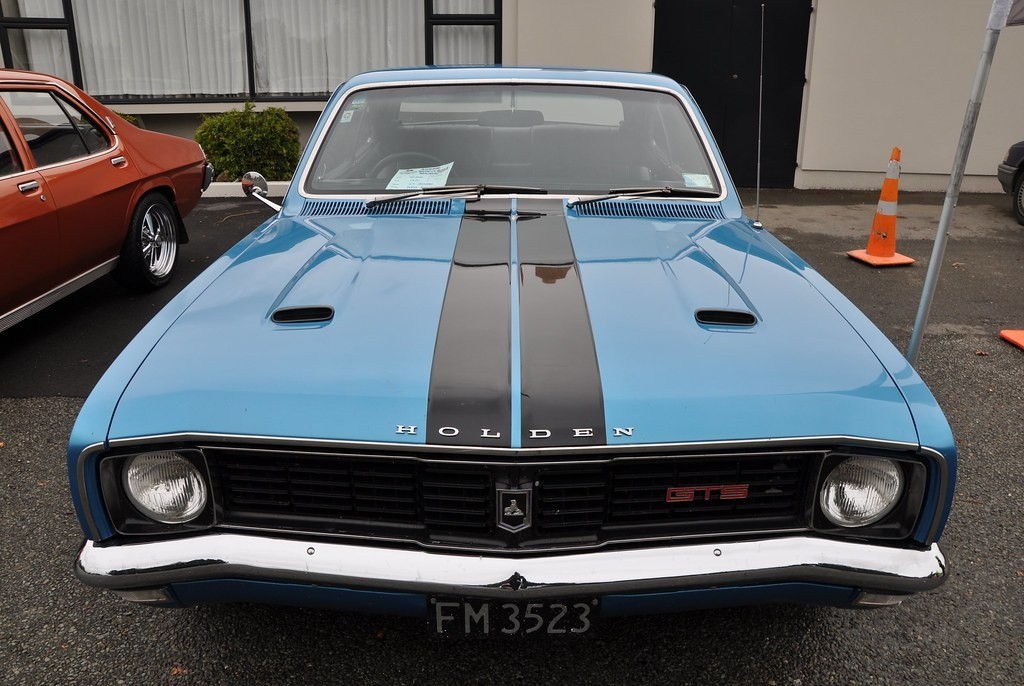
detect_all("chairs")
[531,125,615,183]
[392,125,492,180]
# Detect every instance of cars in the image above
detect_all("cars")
[0,69,215,337]
[996,141,1024,227]
[66,63,959,610]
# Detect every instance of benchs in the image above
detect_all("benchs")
[381,127,633,182]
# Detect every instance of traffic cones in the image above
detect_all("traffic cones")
[846,144,916,268]
